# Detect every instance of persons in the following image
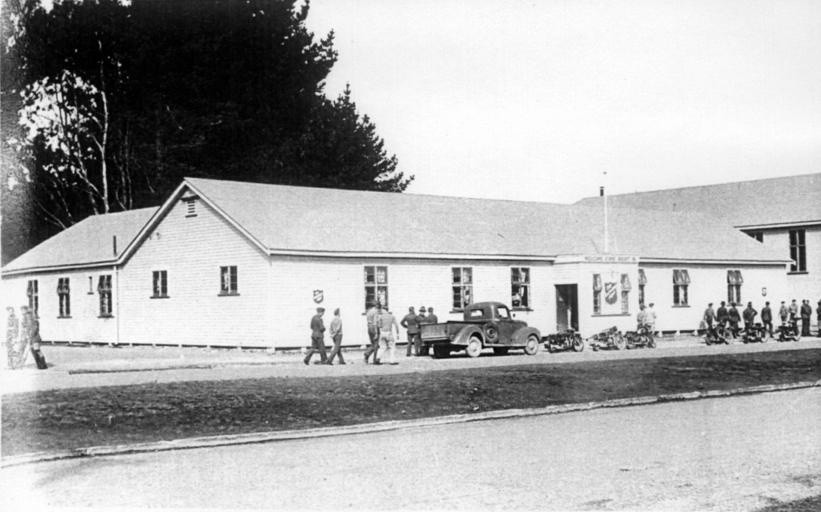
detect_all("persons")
[643,303,657,336]
[417,306,427,357]
[7,307,24,371]
[400,306,422,357]
[704,299,821,338]
[424,306,439,356]
[362,298,383,365]
[21,305,47,368]
[302,307,329,364]
[637,304,647,333]
[375,305,401,365]
[326,307,347,367]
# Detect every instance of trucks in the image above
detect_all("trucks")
[413,300,543,358]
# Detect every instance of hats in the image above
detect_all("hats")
[317,308,325,312]
[420,306,426,312]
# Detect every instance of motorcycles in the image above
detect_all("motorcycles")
[540,325,659,354]
[699,317,804,345]
